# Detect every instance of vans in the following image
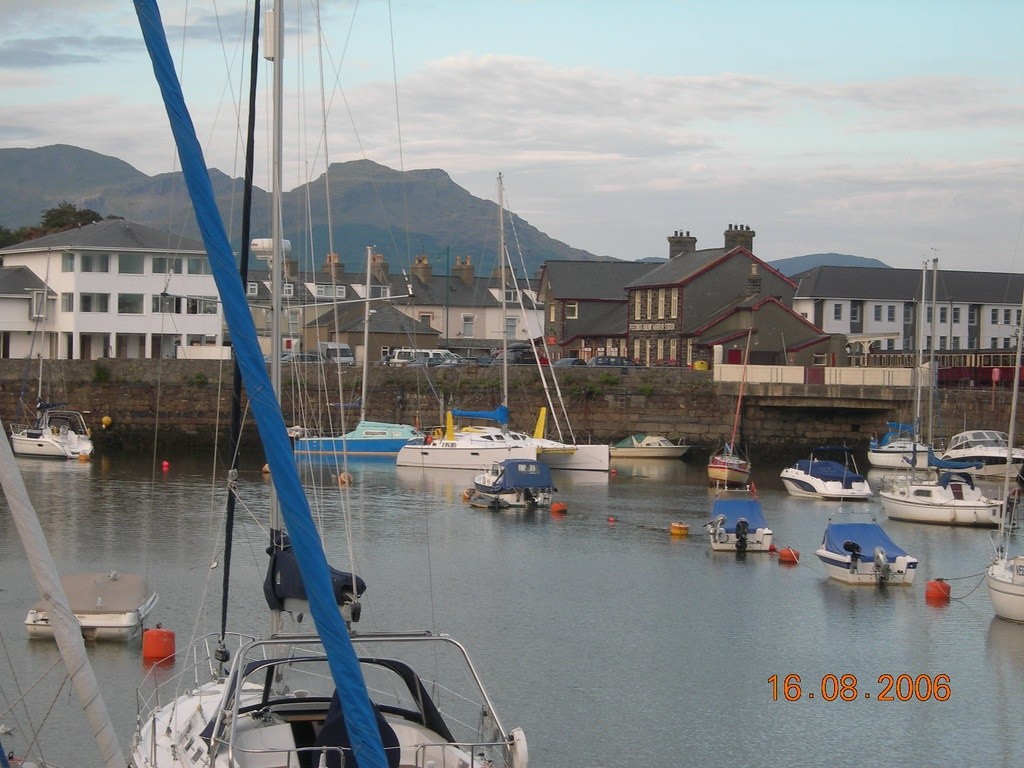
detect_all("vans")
[316,342,354,365]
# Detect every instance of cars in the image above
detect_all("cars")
[376,349,645,370]
[262,351,325,365]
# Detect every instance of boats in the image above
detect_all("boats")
[393,412,538,471]
[816,513,918,585]
[465,456,558,510]
[24,571,159,644]
[780,438,876,502]
[698,478,773,555]
[938,428,1024,478]
[609,433,694,458]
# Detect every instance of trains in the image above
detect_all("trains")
[849,348,1024,386]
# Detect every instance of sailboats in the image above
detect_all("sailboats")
[292,242,428,460]
[11,246,93,459]
[865,261,943,473]
[986,303,1024,625]
[878,253,1004,528]
[457,172,612,472]
[706,328,753,486]
[2,0,528,768]
